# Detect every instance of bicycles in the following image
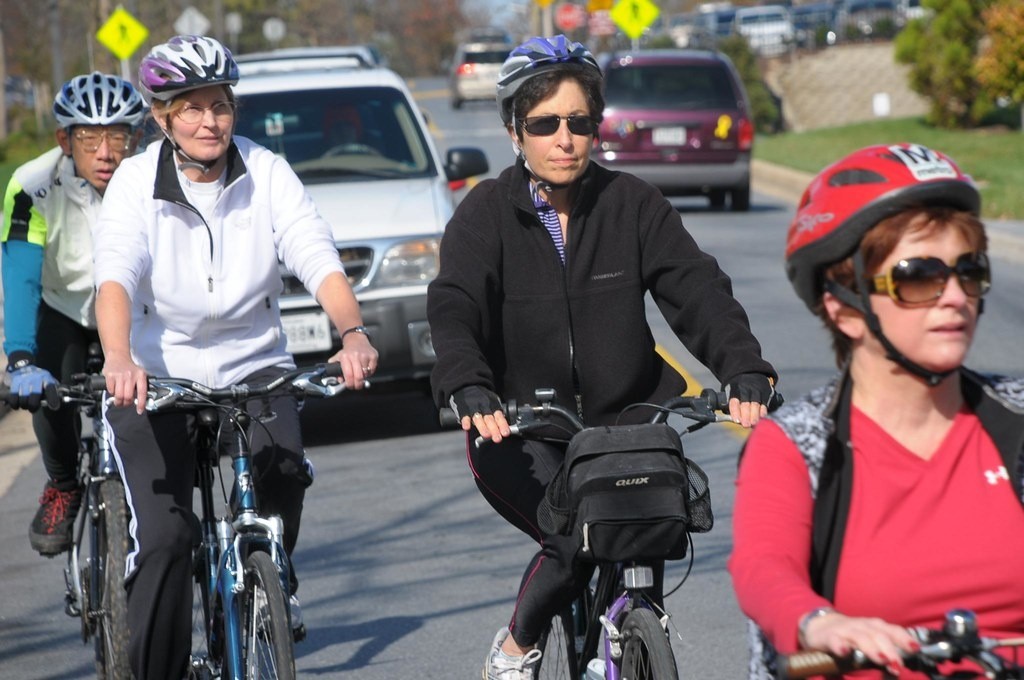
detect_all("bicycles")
[439,388,783,680]
[67,360,374,680]
[0,353,133,680]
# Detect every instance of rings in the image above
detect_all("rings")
[362,367,371,371]
[473,412,482,418]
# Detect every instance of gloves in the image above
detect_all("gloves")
[2,366,61,413]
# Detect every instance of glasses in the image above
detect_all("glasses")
[177,102,237,124]
[518,114,598,136]
[853,250,992,303]
[70,128,135,152]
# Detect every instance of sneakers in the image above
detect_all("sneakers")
[481,627,542,680]
[28,481,86,554]
[250,589,306,644]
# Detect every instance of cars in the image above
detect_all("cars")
[666,1,941,60]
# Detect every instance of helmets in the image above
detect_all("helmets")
[785,143,981,313]
[52,70,152,130]
[138,36,241,105]
[497,34,602,123]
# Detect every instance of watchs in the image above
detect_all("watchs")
[342,325,375,343]
[799,607,840,648]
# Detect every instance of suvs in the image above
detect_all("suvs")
[439,38,520,111]
[581,46,753,210]
[209,41,492,429]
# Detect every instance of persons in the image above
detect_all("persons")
[1,69,147,553]
[90,33,381,680]
[425,32,779,679]
[726,142,1024,680]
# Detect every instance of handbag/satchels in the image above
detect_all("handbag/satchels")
[541,423,712,561]
